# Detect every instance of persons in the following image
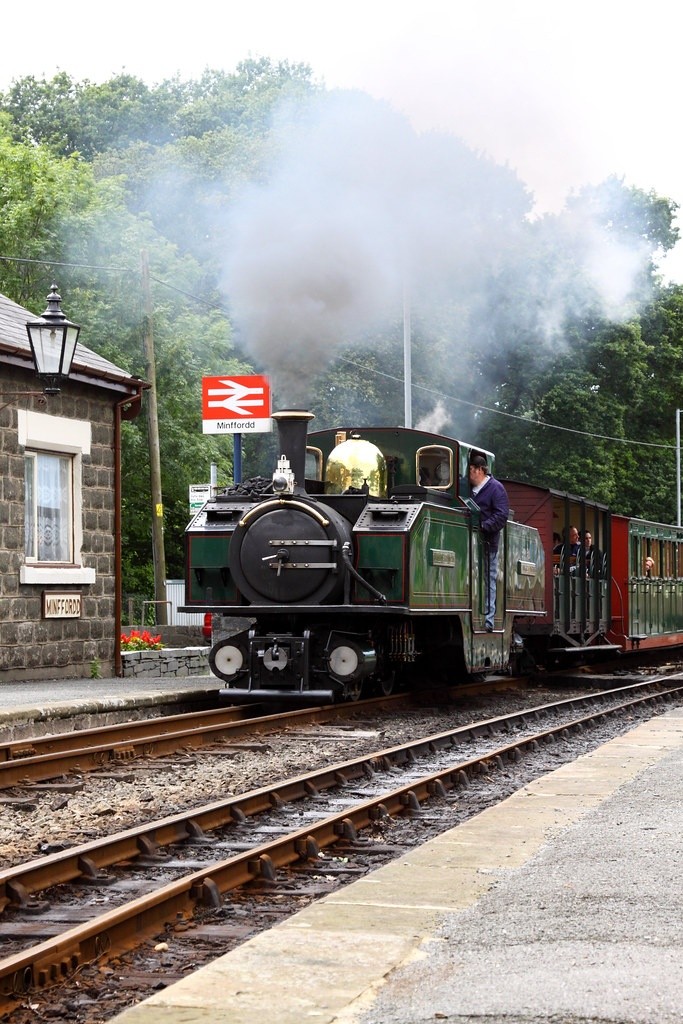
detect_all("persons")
[470,456,510,632]
[646,557,655,576]
[552,526,603,579]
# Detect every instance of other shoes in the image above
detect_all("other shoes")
[485,622,493,633]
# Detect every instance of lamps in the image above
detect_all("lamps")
[26,282,81,394]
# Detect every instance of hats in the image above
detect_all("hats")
[471,455,486,466]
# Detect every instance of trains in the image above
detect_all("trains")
[177,408,683,703]
